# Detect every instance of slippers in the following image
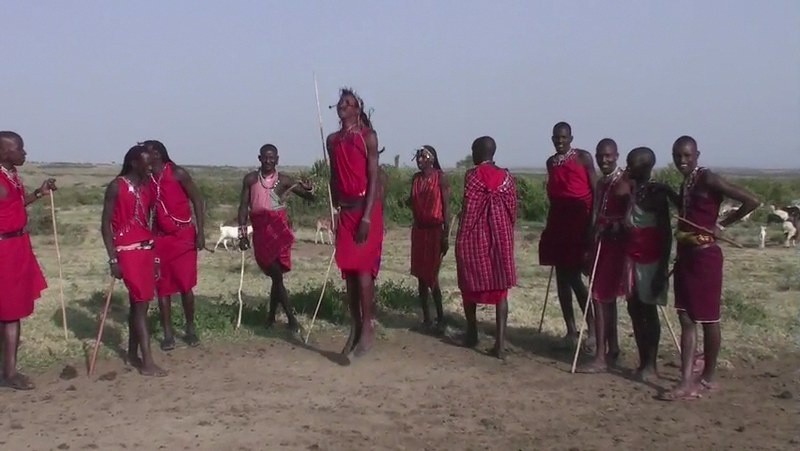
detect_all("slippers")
[690,376,715,400]
[604,349,620,364]
[576,364,609,373]
[658,389,699,401]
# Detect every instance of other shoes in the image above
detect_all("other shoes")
[0,369,34,391]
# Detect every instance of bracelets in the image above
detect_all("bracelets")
[108,258,118,264]
[305,185,314,195]
[443,237,448,241]
[716,223,724,231]
[238,225,247,239]
[362,218,370,224]
[34,189,43,199]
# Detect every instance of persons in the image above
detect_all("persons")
[450,135,517,359]
[325,88,384,367]
[404,145,450,326]
[101,140,205,377]
[0,132,57,390]
[538,122,760,401]
[238,144,314,334]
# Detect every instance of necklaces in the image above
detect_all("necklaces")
[685,166,701,190]
[259,169,280,189]
[551,148,575,166]
[2,167,22,189]
[125,176,143,198]
[602,166,624,183]
[481,160,489,164]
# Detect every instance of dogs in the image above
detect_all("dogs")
[213,224,252,252]
[768,204,800,248]
[313,216,334,246]
